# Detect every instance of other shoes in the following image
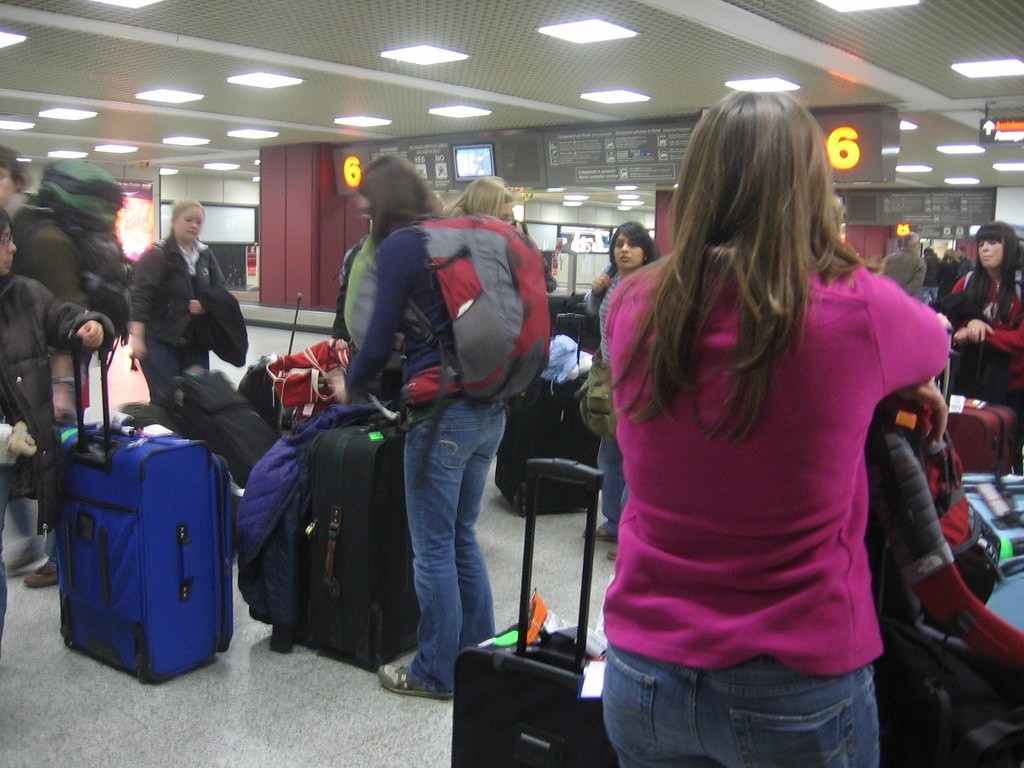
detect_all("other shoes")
[607,546,620,560]
[583,524,617,541]
[24,563,58,588]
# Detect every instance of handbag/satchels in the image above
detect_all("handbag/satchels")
[573,350,617,437]
[239,337,354,434]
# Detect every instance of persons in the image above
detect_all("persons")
[583,222,656,561]
[330,157,558,698]
[923,248,974,299]
[0,205,115,587]
[881,234,926,299]
[129,201,223,410]
[952,221,1024,475]
[603,90,950,768]
[0,146,91,576]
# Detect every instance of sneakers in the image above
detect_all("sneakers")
[378,664,454,699]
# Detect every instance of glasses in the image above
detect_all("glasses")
[0,236,14,246]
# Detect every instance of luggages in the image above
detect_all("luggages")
[293,424,420,671]
[451,457,619,768]
[54,327,234,685]
[935,329,1018,477]
[495,313,601,516]
[159,364,278,491]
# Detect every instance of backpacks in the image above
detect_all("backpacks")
[868,391,1024,661]
[21,157,132,346]
[386,212,550,409]
